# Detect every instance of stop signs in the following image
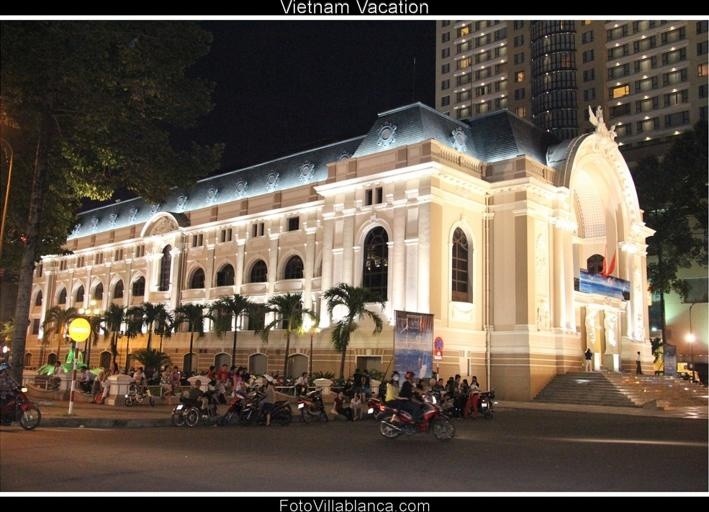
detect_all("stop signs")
[68,317,91,343]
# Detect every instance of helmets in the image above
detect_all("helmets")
[405,371,416,379]
[263,371,274,382]
[0,362,11,371]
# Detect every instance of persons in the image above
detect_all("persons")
[37,359,482,425]
[0,361,24,425]
[584,344,594,373]
[634,350,644,376]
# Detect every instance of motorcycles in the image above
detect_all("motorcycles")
[0,363,42,430]
